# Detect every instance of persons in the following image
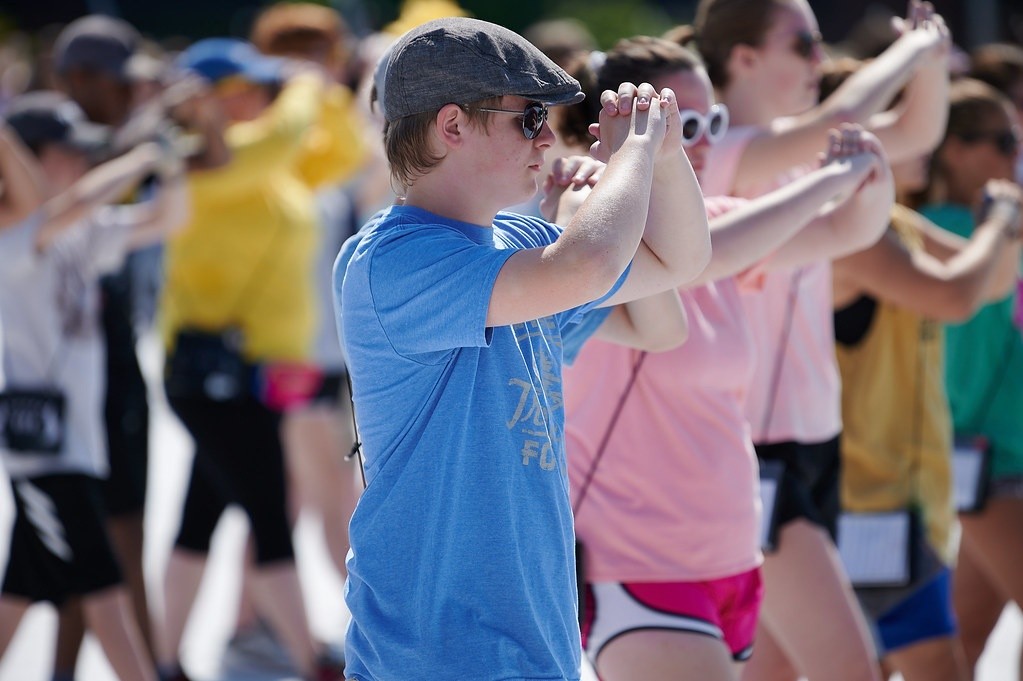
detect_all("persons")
[548,35,896,681]
[0,91,191,681]
[49,1,601,681]
[331,17,713,681]
[665,0,947,681]
[814,42,1023,681]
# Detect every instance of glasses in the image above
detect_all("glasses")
[755,31,824,66]
[960,128,1017,158]
[458,101,550,139]
[675,103,729,148]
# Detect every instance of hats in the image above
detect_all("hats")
[258,5,354,75]
[173,40,291,94]
[373,15,587,117]
[53,16,163,81]
[5,92,106,152]
